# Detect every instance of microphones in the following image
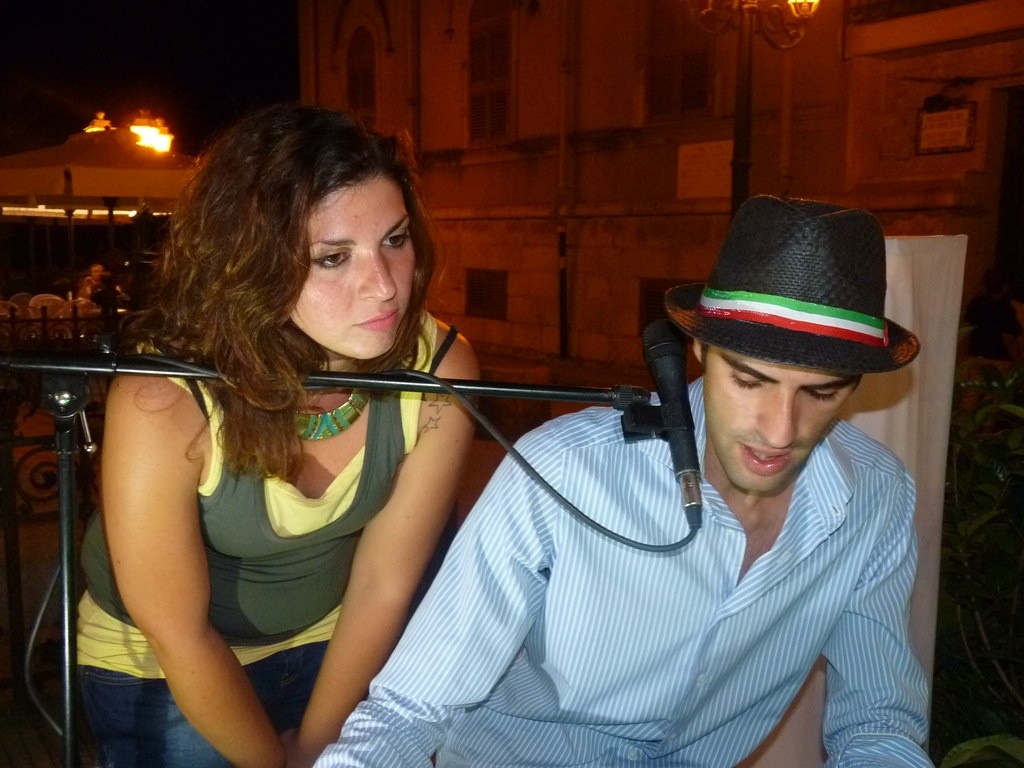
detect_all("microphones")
[642,318,703,527]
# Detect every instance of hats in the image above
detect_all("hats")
[665,193,920,372]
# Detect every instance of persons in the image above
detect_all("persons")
[78,264,121,299]
[311,194,937,766]
[78,105,481,768]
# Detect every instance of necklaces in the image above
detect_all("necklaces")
[293,388,370,441]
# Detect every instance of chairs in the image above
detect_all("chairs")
[0,291,129,343]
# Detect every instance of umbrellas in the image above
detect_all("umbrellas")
[0,130,199,275]
